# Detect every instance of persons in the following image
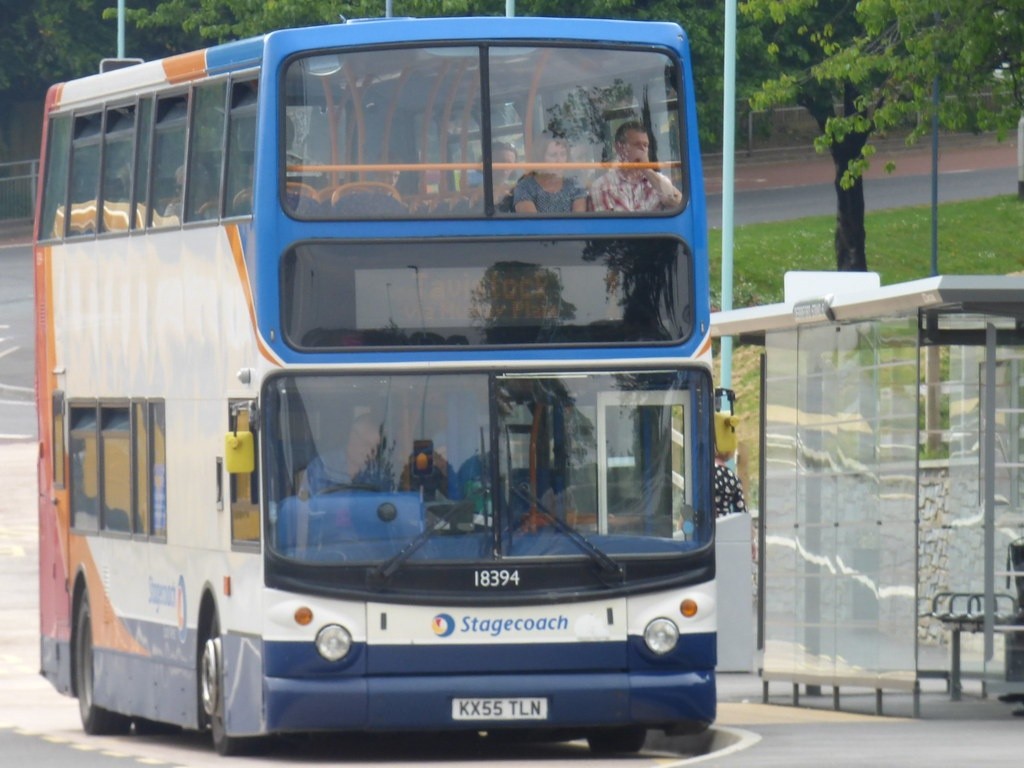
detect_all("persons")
[470,141,517,206]
[163,158,214,223]
[374,150,405,202]
[591,120,684,212]
[514,131,589,214]
[294,419,381,556]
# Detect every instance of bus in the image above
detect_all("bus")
[33,18,738,758]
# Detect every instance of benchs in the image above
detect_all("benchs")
[918,592,1024,702]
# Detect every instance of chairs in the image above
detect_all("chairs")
[156,180,594,219]
